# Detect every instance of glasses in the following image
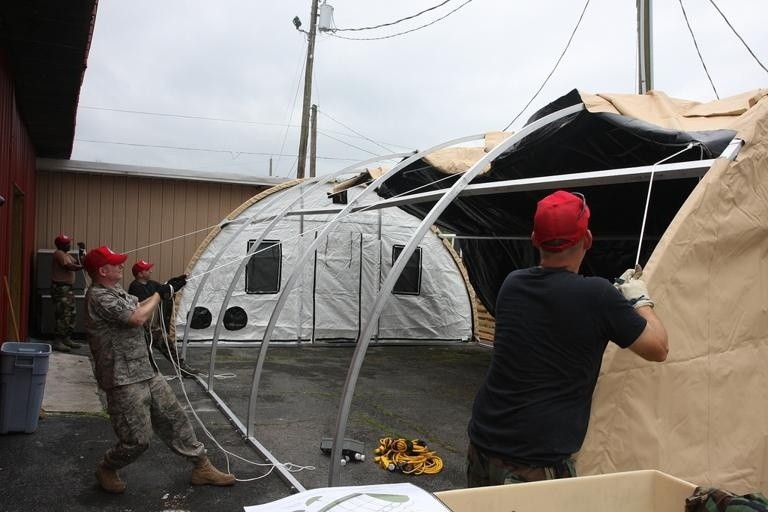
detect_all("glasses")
[570,191,586,221]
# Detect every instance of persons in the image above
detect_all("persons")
[466,191,668,489]
[83,246,236,493]
[128,261,202,379]
[52,235,86,352]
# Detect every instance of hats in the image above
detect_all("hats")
[55,235,73,247]
[84,246,127,273]
[132,261,153,275]
[534,191,590,252]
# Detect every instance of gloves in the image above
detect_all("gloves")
[613,268,654,309]
[157,274,186,300]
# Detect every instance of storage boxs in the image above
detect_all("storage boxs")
[430,468,697,512]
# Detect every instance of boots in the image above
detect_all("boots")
[191,455,235,486]
[53,338,70,351]
[63,336,81,349]
[179,360,199,376]
[95,459,125,493]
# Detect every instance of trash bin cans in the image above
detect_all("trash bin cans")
[0,342,52,434]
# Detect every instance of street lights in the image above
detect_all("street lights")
[292,0,318,180]
